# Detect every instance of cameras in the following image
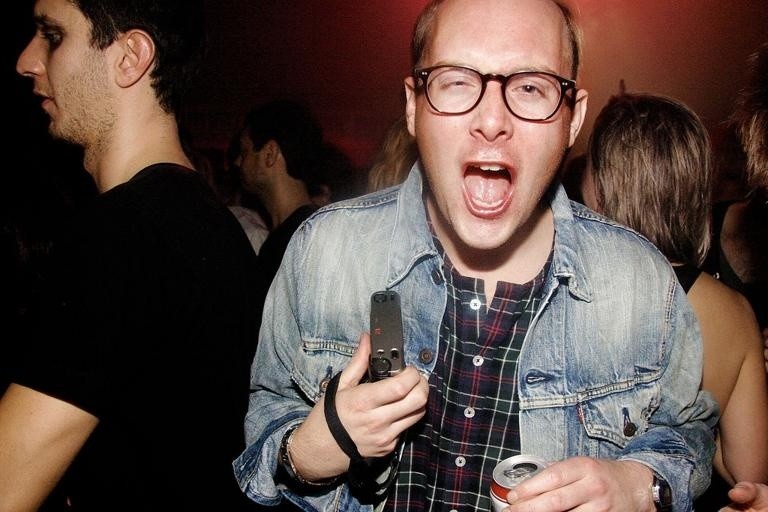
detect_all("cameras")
[367,291,403,383]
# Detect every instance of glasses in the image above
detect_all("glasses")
[414,64,576,121]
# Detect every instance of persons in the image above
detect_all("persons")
[234,0,719,512]
[584,92,766,512]
[2,2,268,512]
[721,43,766,328]
[365,115,420,195]
[178,99,354,279]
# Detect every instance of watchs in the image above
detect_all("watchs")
[652,471,673,512]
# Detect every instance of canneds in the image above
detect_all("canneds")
[490,454,549,512]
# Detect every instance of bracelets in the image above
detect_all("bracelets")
[281,421,338,487]
